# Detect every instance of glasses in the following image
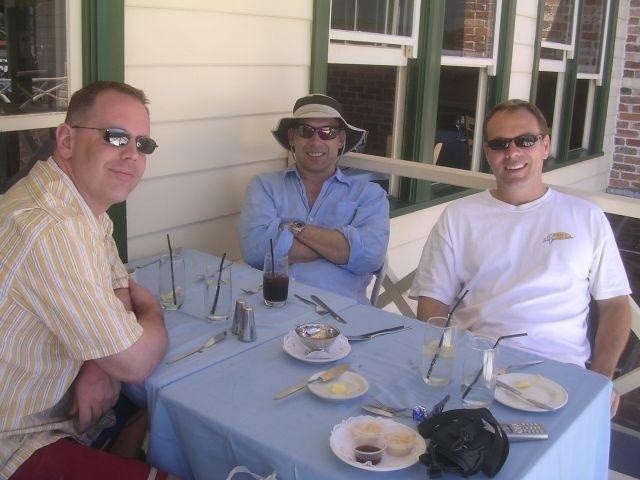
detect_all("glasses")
[484,132,550,150]
[296,124,342,140]
[72,126,158,154]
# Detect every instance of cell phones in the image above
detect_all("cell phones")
[498,423,548,443]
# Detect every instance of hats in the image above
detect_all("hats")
[271,94,366,156]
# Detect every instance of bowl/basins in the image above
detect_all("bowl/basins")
[295,324,341,353]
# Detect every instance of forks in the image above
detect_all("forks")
[240,282,262,295]
[373,400,422,414]
[294,293,333,319]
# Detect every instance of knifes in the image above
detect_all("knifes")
[313,293,350,328]
[344,321,408,343]
[165,329,229,366]
[501,380,557,412]
[272,363,351,402]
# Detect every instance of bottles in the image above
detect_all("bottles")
[231,298,256,343]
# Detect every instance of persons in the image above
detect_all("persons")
[238,92,390,305]
[408,99,633,419]
[0,82,177,480]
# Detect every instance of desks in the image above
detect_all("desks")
[109,250,360,415]
[148,301,613,478]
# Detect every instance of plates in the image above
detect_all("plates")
[281,335,352,362]
[306,369,370,399]
[494,372,569,413]
[328,417,425,471]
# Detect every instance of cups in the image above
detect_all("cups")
[158,252,188,311]
[384,426,419,457]
[420,317,458,387]
[461,336,500,412]
[123,267,138,285]
[206,265,233,321]
[350,434,387,465]
[262,252,290,309]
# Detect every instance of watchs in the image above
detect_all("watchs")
[291,220,306,234]
